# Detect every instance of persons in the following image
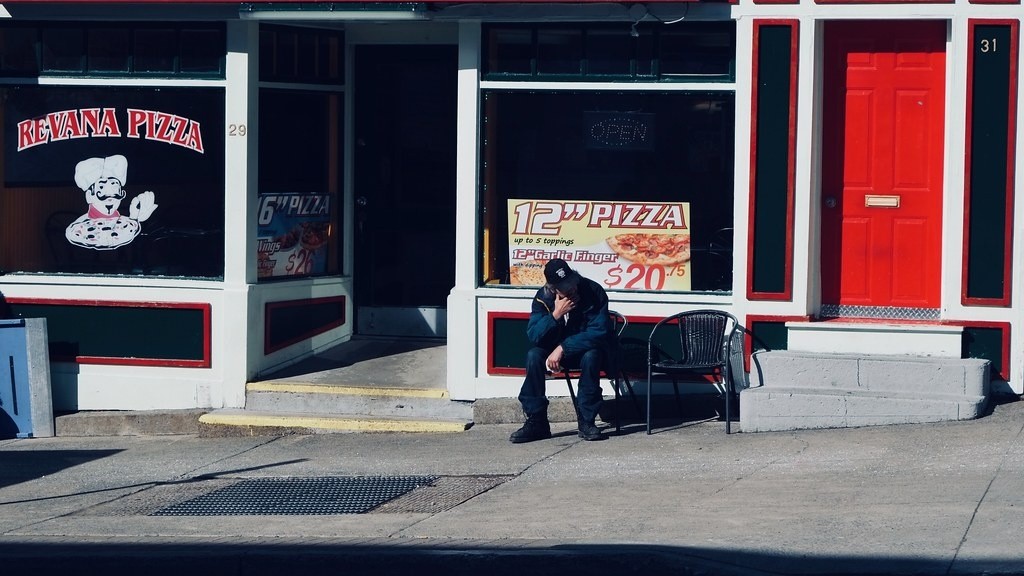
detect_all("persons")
[73,153,158,222]
[508,257,621,443]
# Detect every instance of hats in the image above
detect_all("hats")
[544,259,579,293]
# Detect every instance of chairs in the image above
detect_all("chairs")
[647,307,739,438]
[540,309,638,434]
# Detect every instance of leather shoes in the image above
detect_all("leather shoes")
[508,413,553,443]
[577,418,601,441]
